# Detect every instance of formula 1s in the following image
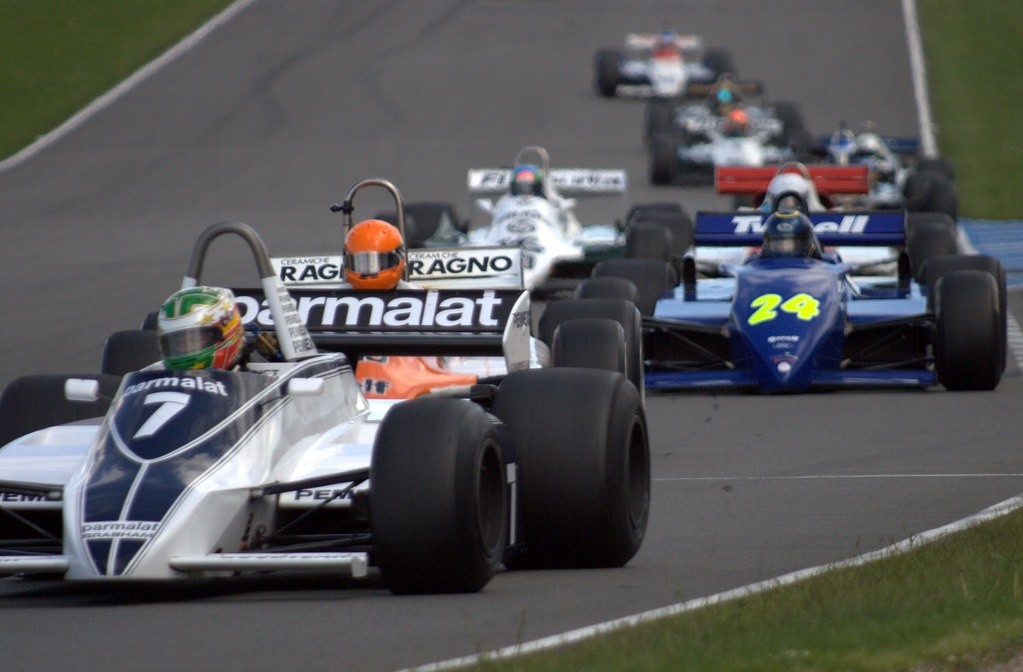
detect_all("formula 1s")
[0,217,657,593]
[231,31,1009,414]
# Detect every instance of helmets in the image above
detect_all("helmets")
[715,88,733,104]
[341,218,406,290]
[508,164,543,196]
[730,110,748,125]
[851,131,887,159]
[156,285,246,370]
[657,28,676,45]
[766,172,810,212]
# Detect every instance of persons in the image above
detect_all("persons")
[339,220,419,289]
[501,164,551,200]
[141,286,267,376]
[742,209,838,265]
